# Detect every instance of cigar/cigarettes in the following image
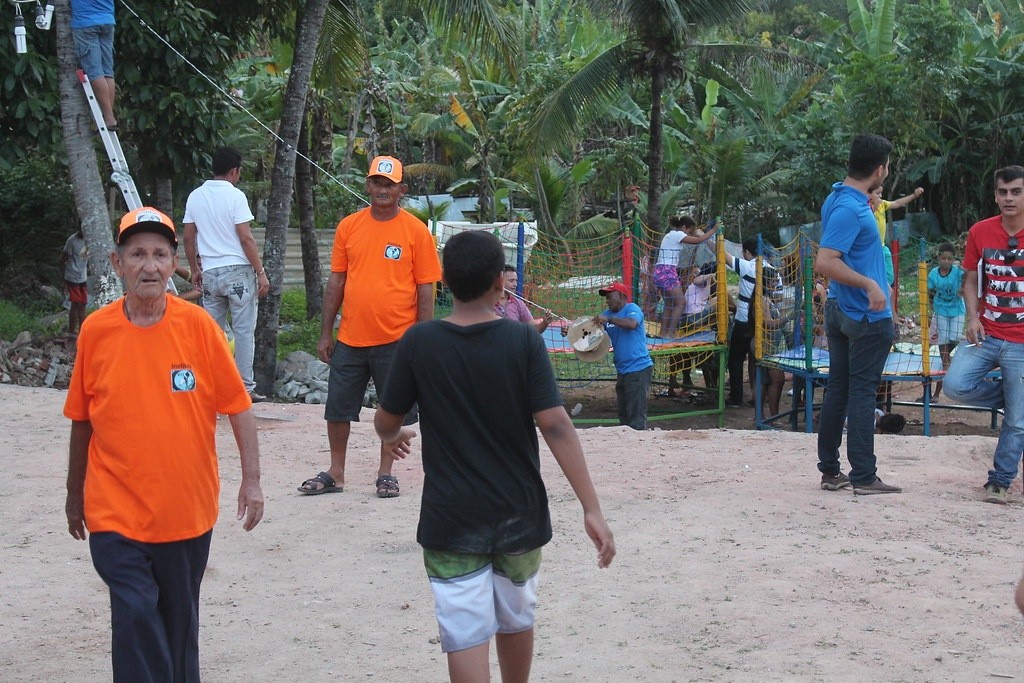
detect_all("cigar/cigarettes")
[966,342,982,347]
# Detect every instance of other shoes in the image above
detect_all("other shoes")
[249,391,266,404]
[788,388,793,396]
[915,395,938,403]
[744,398,756,405]
[725,399,739,407]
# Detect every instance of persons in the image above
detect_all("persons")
[174,253,235,357]
[492,264,553,334]
[815,132,903,495]
[183,147,270,403]
[927,244,964,371]
[375,230,616,683]
[561,283,655,431]
[654,216,718,398]
[696,228,823,427]
[59,220,89,350]
[297,155,443,497]
[62,207,264,683]
[868,183,924,245]
[70,0,117,131]
[943,165,1024,504]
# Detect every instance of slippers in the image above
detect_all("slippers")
[297,471,344,495]
[90,123,119,135]
[375,476,399,497]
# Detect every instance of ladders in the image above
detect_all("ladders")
[77,67,179,299]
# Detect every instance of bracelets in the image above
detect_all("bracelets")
[186,270,191,280]
[259,268,265,274]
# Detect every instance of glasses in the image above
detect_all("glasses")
[1004,236,1019,265]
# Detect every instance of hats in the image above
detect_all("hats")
[366,156,407,183]
[598,283,631,299]
[117,206,179,252]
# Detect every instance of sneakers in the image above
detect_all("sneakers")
[982,481,1006,503]
[821,470,850,490]
[852,476,902,494]
